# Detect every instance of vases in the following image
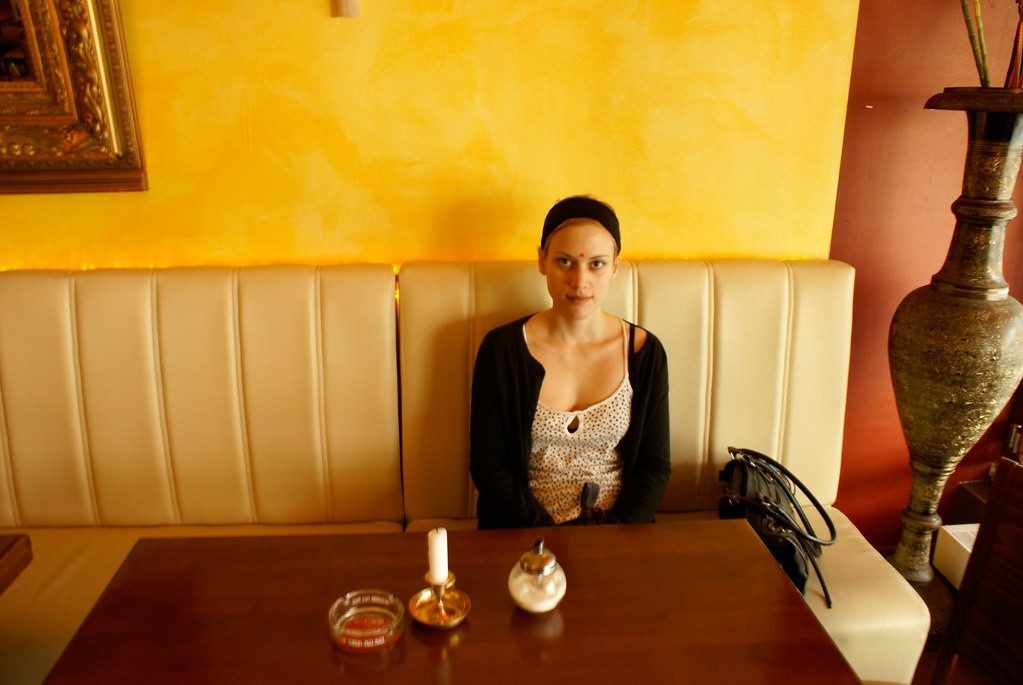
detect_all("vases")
[887,87,1023,582]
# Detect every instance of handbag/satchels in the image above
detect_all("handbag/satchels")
[717,448,837,610]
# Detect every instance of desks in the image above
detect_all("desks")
[46,519,863,685]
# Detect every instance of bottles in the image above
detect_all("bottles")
[508,538,567,613]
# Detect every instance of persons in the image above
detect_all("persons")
[469,194,674,528]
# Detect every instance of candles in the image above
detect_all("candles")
[427,525,449,583]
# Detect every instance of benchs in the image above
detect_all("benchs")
[0,256,935,685]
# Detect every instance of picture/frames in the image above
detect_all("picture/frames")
[0,0,148,195]
